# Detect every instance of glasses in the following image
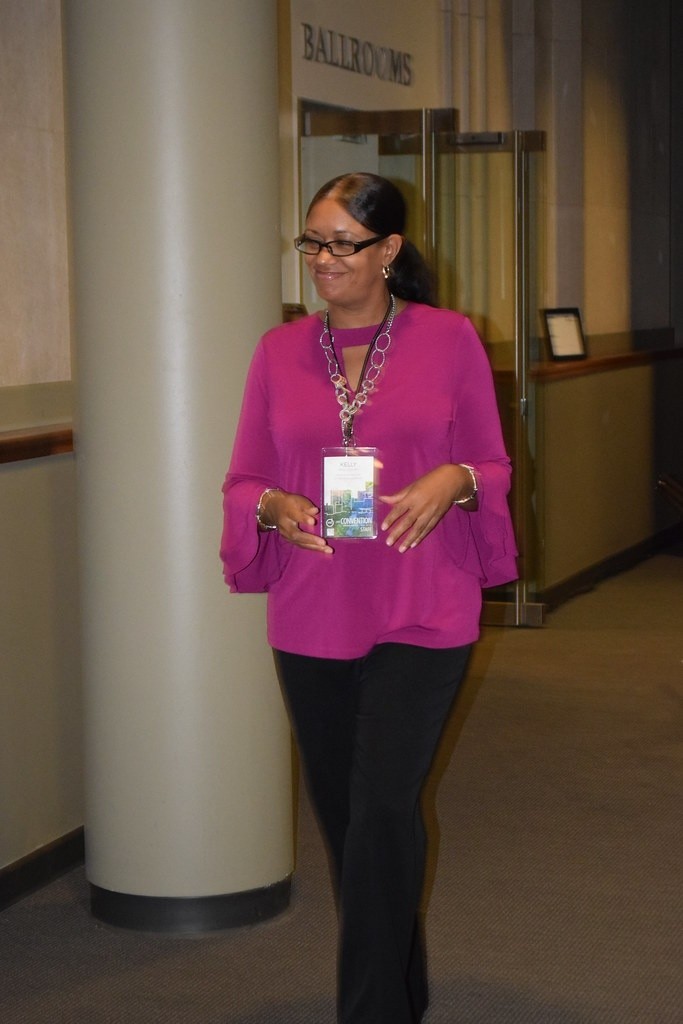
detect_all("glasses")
[293,234,394,257]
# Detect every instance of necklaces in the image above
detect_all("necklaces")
[320,295,397,450]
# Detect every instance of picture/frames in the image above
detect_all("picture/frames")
[537,306,589,361]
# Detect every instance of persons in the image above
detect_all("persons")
[217,168,520,1024]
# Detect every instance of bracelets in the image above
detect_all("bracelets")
[256,489,279,529]
[453,463,479,504]
